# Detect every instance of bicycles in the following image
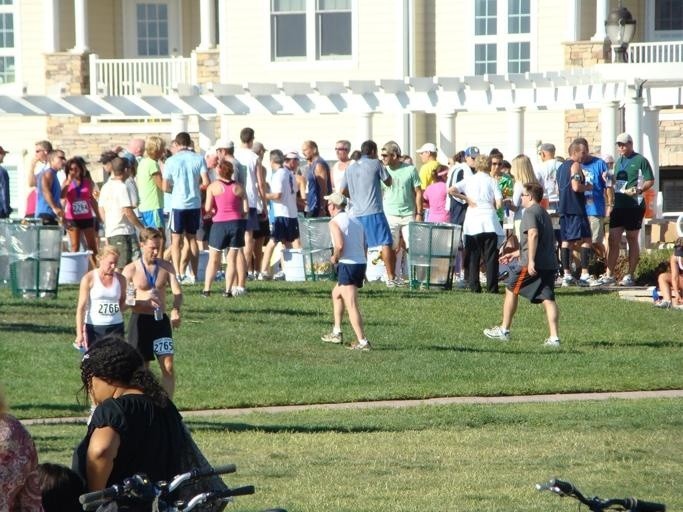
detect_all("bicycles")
[534,477,669,511]
[78,466,289,512]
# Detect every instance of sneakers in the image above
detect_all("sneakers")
[484,324,511,342]
[321,330,344,345]
[175,268,285,297]
[454,270,676,299]
[541,336,561,347]
[344,338,374,354]
[386,273,410,288]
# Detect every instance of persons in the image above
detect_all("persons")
[0,410,43,512]
[38,463,85,512]
[320,193,373,352]
[483,182,561,348]
[72,337,194,512]
[121,228,182,402]
[74,245,136,355]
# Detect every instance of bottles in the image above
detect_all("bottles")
[605,168,613,187]
[126,282,136,306]
[153,296,163,320]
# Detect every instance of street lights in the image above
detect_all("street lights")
[604,7,635,135]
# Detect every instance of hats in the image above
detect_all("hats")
[615,132,633,145]
[465,146,480,159]
[0,147,9,154]
[416,142,438,154]
[211,138,236,151]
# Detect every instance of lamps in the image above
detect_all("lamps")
[603,0,637,65]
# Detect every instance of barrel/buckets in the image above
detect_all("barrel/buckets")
[280,248,306,282]
[642,187,656,218]
[188,250,209,282]
[57,250,94,285]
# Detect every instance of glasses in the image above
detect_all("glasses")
[492,162,503,166]
[54,155,67,160]
[381,154,390,157]
[335,147,347,152]
